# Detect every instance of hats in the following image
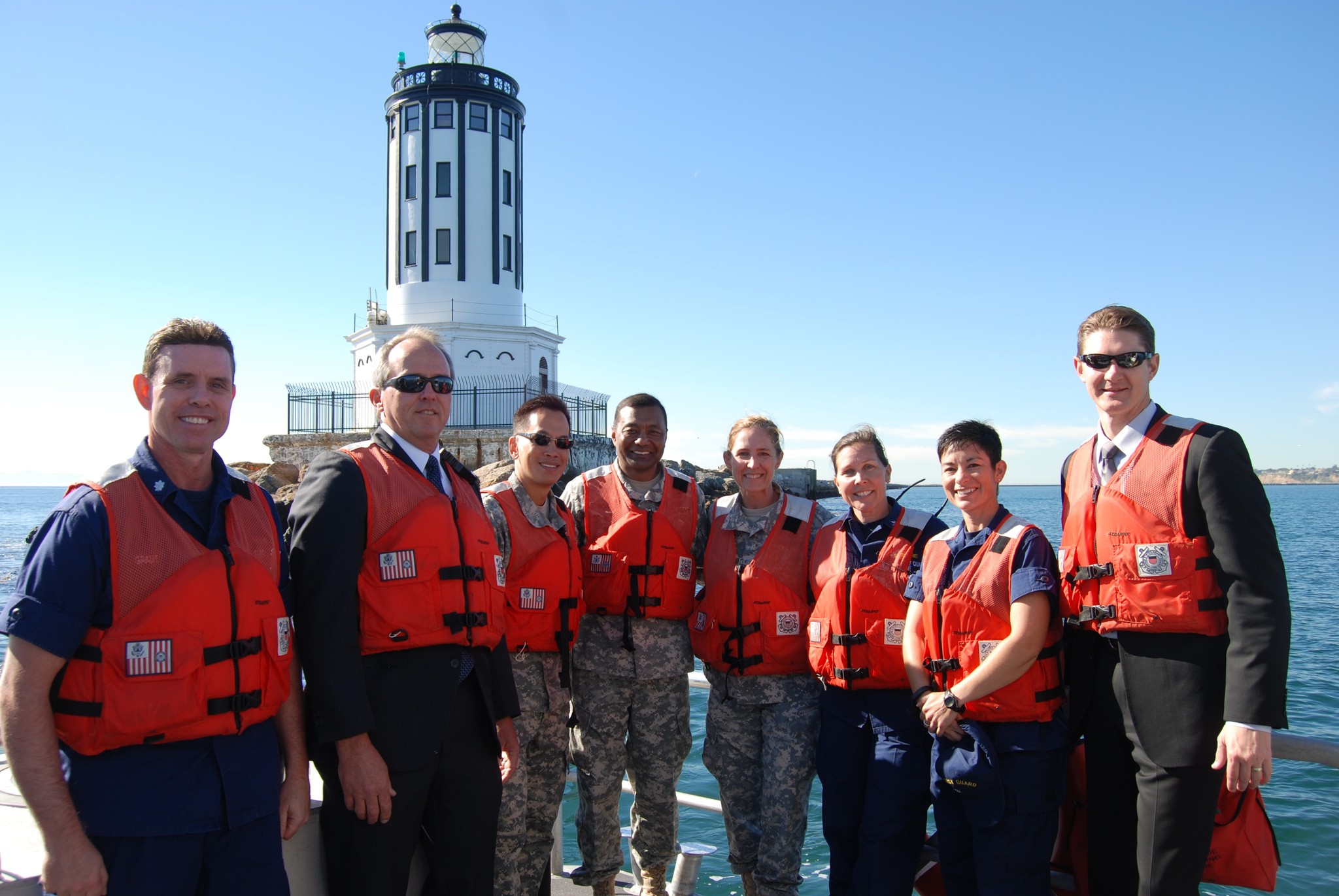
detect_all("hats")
[930,718,1004,822]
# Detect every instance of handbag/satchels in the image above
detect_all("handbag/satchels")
[1049,738,1283,891]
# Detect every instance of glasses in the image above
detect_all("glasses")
[515,432,575,450]
[379,373,454,395]
[1078,352,1153,370]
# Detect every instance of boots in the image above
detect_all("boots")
[591,874,615,896]
[641,872,669,896]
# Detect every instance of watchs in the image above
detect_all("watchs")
[944,689,965,714]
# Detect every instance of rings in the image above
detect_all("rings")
[1253,768,1262,771]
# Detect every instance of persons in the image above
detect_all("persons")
[689,415,836,896]
[806,424,950,896]
[902,418,1060,896]
[480,395,583,896]
[559,394,705,896]
[289,325,522,896]
[0,318,310,896]
[1058,307,1292,896]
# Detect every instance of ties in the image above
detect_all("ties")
[423,454,445,493]
[1100,442,1119,484]
